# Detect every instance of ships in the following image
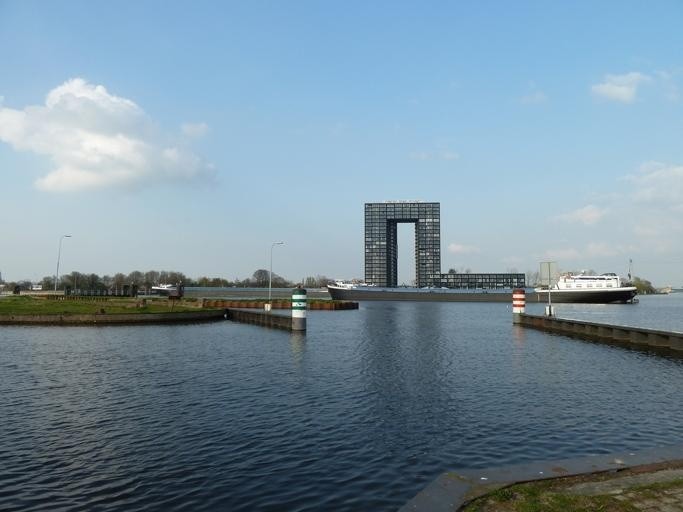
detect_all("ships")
[324,269,639,304]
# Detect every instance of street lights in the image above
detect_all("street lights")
[268,242,284,304]
[54,234,72,290]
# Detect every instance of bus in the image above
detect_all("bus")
[28,284,43,291]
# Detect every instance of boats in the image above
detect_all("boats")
[151,283,184,300]
